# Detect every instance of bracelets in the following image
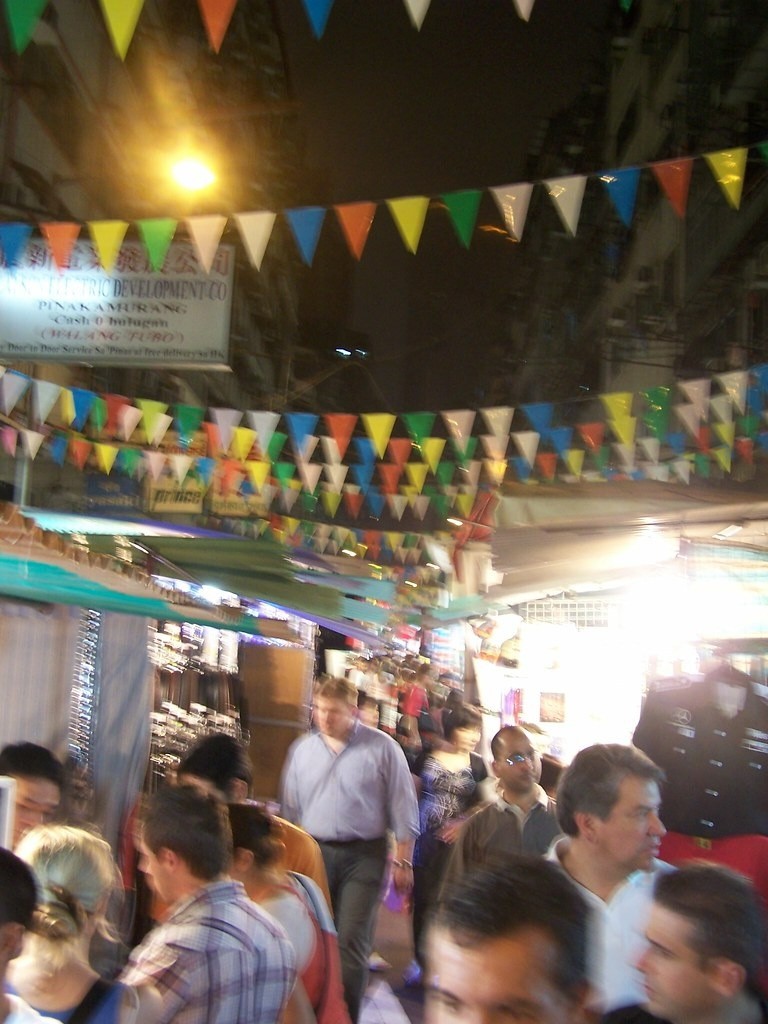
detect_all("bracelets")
[390,857,414,869]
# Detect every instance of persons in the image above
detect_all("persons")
[346,644,768,1024]
[0,731,354,1024]
[280,675,419,1023]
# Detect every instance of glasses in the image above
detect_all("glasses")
[506,748,540,766]
